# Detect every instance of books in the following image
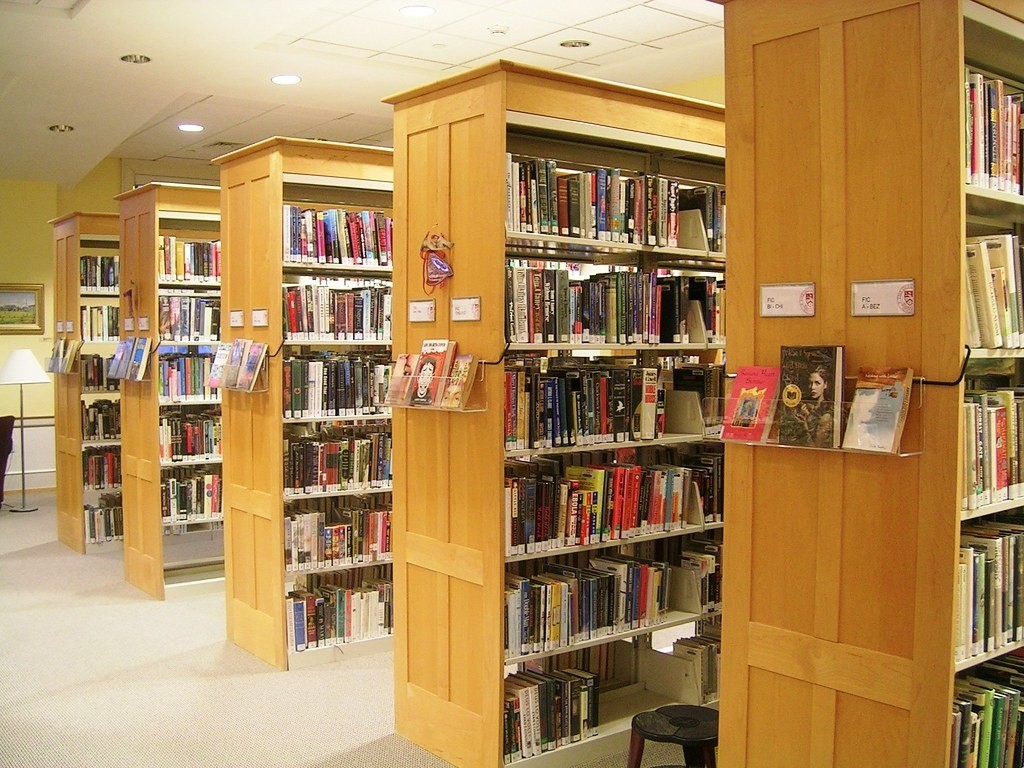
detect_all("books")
[503,151,727,764]
[107,336,152,382]
[385,339,479,409]
[206,338,268,392]
[282,204,393,653]
[48,338,79,374]
[949,70,1024,768]
[158,235,223,535]
[720,345,913,456]
[79,255,123,544]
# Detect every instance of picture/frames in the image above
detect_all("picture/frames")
[0,282,45,336]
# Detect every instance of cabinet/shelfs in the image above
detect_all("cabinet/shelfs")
[717,0,1024,767]
[380,54,727,768]
[45,134,395,674]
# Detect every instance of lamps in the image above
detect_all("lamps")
[0,349,51,513]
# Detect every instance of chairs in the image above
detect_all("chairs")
[625,704,718,767]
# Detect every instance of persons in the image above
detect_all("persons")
[791,366,833,448]
